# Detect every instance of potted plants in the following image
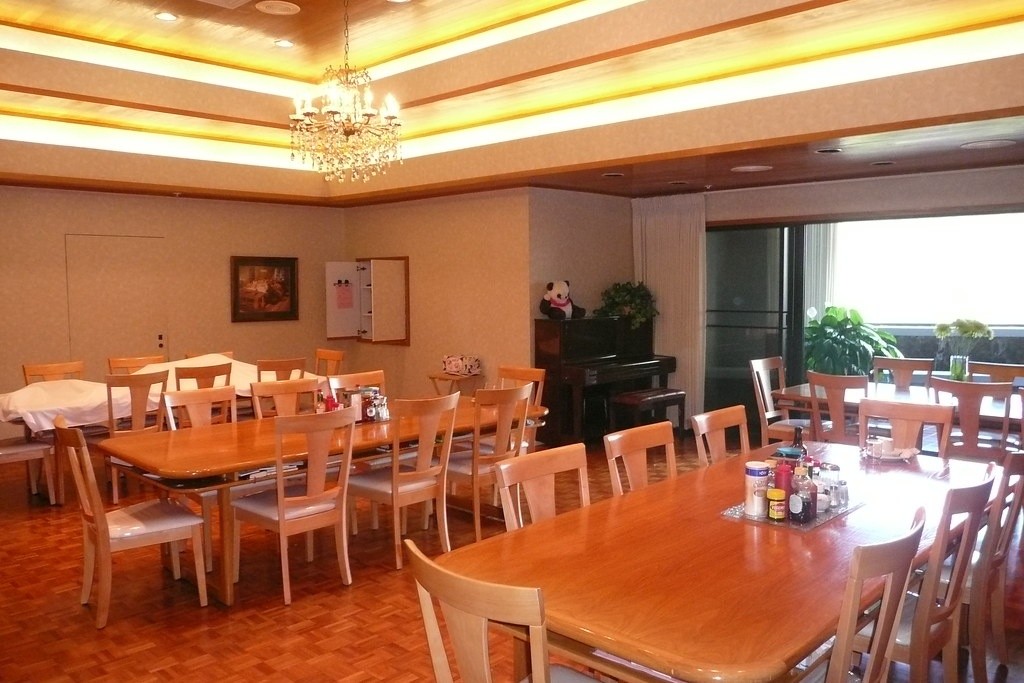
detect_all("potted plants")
[593,280,660,329]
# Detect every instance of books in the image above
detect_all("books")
[373,432,473,455]
[238,461,303,479]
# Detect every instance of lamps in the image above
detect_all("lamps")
[287,1,406,182]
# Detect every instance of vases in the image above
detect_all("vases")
[950,355,969,381]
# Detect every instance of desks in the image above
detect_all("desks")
[435,440,1021,683]
[428,371,481,396]
[100,395,550,607]
[771,382,1024,448]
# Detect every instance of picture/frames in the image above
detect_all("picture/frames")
[230,256,299,322]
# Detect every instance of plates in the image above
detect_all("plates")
[867,448,920,461]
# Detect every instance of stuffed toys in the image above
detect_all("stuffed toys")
[539,280,586,320]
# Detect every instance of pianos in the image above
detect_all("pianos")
[533,314,677,448]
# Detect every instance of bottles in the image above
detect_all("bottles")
[764,426,848,523]
[315,384,389,424]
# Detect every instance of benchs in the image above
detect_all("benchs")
[607,387,686,442]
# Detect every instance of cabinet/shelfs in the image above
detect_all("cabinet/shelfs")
[324,257,411,348]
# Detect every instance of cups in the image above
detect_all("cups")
[879,437,894,452]
[753,490,766,513]
[866,439,882,458]
[745,461,768,516]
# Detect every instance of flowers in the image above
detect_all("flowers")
[933,319,994,380]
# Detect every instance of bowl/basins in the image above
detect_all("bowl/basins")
[817,494,831,512]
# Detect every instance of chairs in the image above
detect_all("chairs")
[405,356,1024,683]
[0,349,545,629]
